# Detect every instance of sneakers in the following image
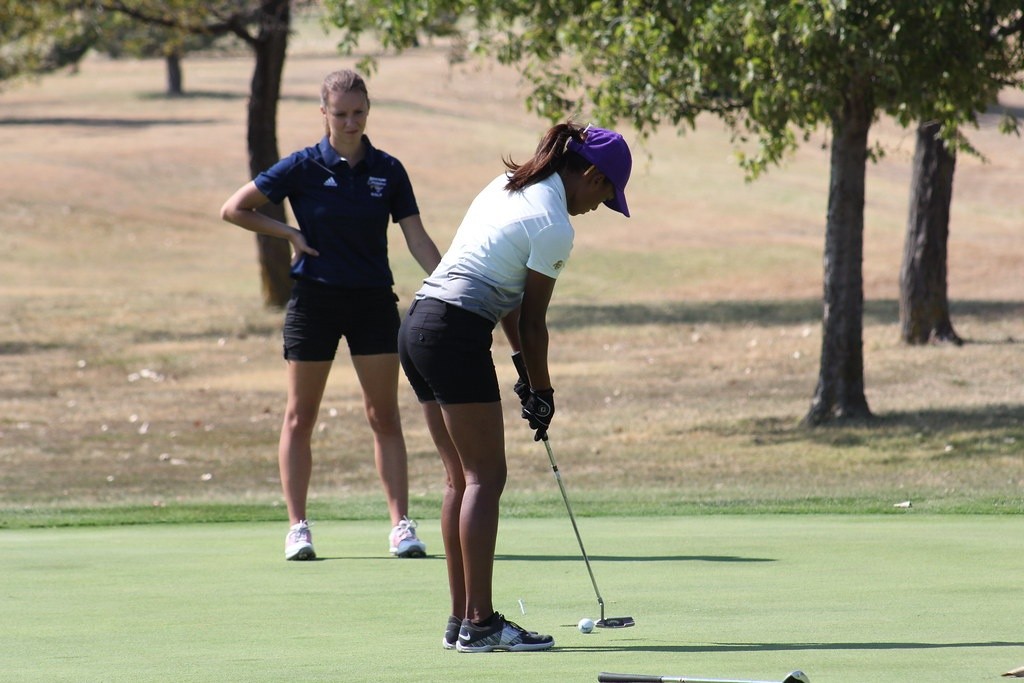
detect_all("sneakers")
[443,613,462,652]
[286,520,316,561]
[388,514,428,557]
[455,615,556,652]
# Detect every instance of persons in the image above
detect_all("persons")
[396,122,631,652]
[221,71,440,559]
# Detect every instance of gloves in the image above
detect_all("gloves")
[514,376,530,409]
[520,387,555,442]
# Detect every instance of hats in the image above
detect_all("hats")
[567,128,631,219]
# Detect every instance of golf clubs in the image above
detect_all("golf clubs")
[510,350,636,627]
[597,670,810,683]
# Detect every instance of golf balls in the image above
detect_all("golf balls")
[578,618,594,633]
